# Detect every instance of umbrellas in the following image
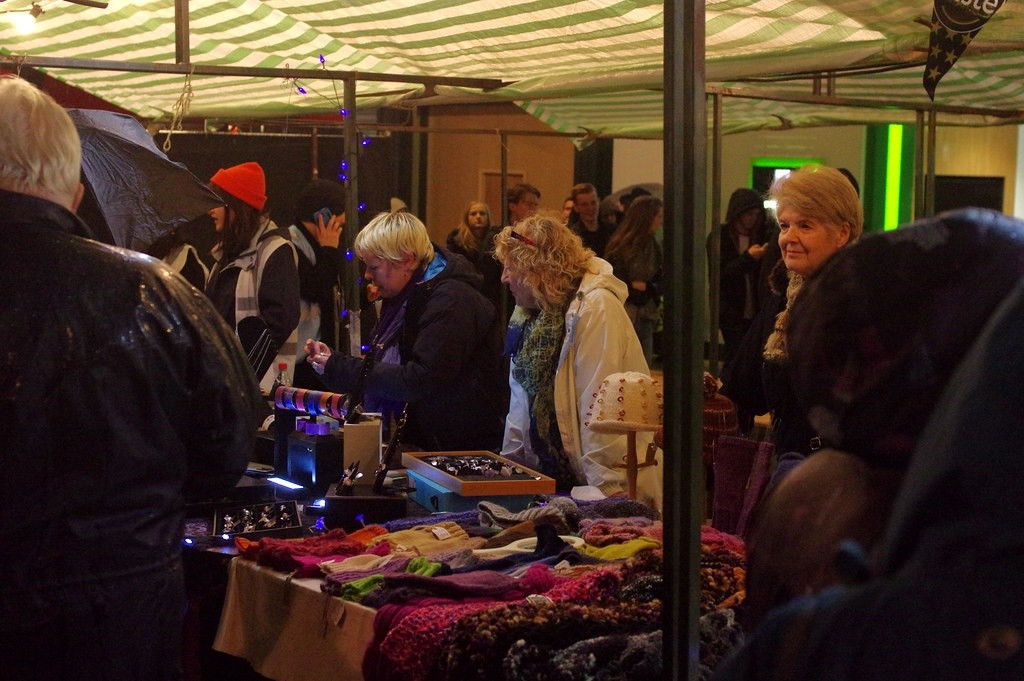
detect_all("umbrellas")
[62,107,228,254]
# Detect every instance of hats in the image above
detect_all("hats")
[584,371,664,434]
[210,162,267,211]
[654,372,742,465]
[238,499,665,594]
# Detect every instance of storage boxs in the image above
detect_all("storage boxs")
[213,501,303,546]
[325,483,405,535]
[343,421,382,485]
[402,450,556,495]
[406,470,546,517]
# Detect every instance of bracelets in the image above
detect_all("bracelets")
[275,386,343,418]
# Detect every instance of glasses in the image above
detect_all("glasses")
[502,226,547,252]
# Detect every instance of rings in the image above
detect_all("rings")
[313,363,317,368]
[317,356,320,359]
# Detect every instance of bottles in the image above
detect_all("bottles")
[278,362,292,387]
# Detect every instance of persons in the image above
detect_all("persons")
[445,183,664,365]
[201,162,301,385]
[0,73,274,681]
[493,216,664,512]
[286,178,346,387]
[306,210,505,451]
[705,162,863,442]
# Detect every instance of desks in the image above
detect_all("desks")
[185,546,239,681]
[213,563,378,681]
